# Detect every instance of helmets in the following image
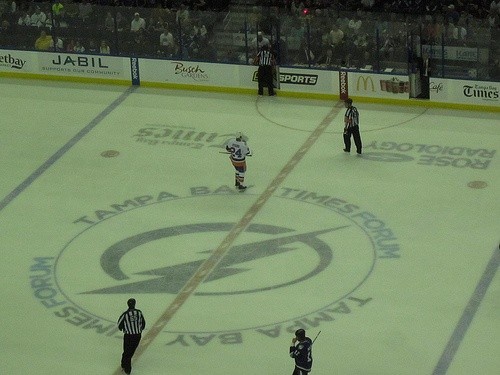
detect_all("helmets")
[296,328,307,338]
[235,131,244,138]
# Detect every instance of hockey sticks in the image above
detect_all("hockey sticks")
[312,329,322,344]
[219,152,251,157]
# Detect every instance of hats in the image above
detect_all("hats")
[344,98,353,103]
[258,32,263,35]
[134,12,139,15]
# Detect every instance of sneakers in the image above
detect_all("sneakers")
[234,182,240,188]
[356,150,363,156]
[269,92,276,97]
[238,184,248,192]
[257,91,263,97]
[343,147,351,153]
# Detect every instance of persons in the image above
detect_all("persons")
[289,329,313,375]
[226,132,250,191]
[343,98,363,154]
[0,0,217,63]
[255,45,276,96]
[223,0,500,81]
[117,298,145,375]
